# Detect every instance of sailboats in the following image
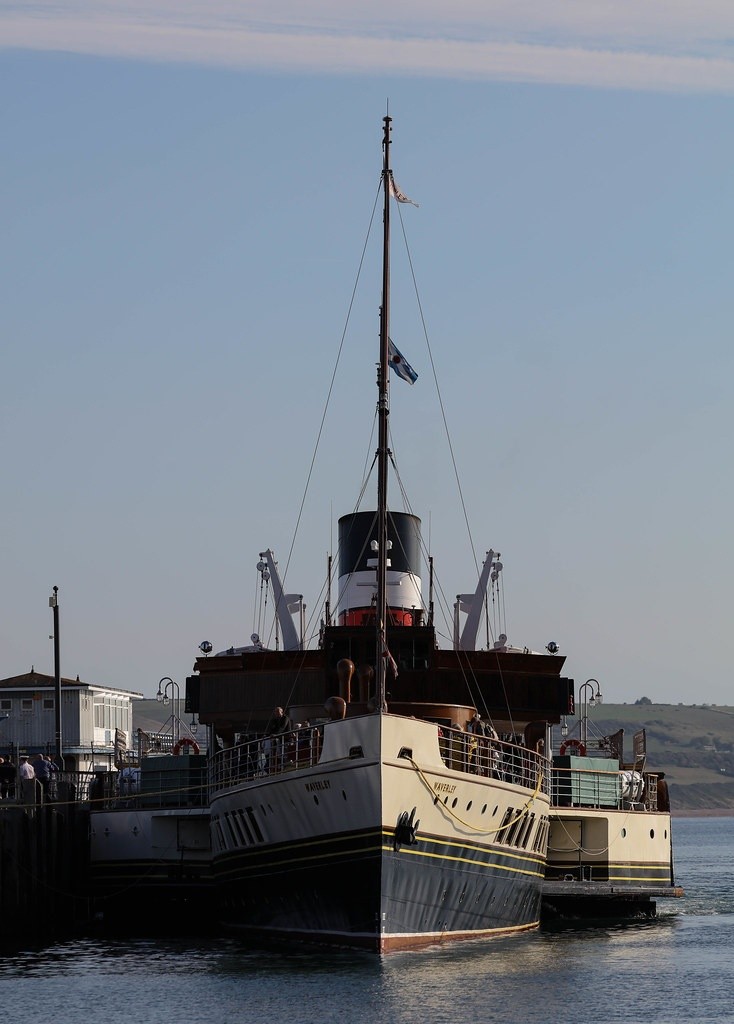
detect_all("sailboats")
[1,108,682,974]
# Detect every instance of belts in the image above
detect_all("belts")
[24,779,33,780]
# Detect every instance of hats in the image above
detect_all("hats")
[19,756,29,759]
[0,758,4,764]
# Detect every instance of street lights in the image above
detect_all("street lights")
[578,683,597,754]
[154,676,176,752]
[162,681,180,743]
[583,679,603,755]
[47,585,69,780]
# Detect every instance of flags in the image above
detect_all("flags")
[388,337,419,385]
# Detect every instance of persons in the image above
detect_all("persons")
[438,713,546,792]
[261,706,378,779]
[0,752,59,803]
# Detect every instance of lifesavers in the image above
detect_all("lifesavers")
[173,738,201,755]
[560,739,587,757]
